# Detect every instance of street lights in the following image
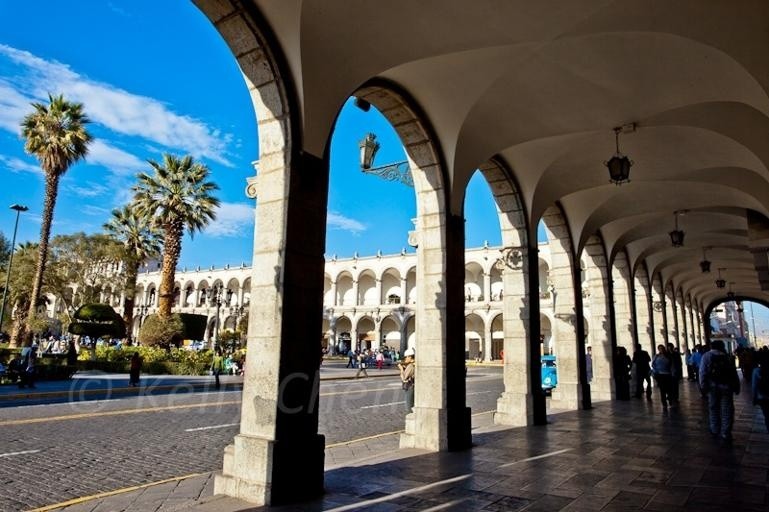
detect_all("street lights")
[205,282,233,356]
[137,304,148,346]
[0,204,29,334]
[229,302,244,353]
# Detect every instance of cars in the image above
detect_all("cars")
[540,355,557,393]
[184,340,209,353]
[60,334,119,347]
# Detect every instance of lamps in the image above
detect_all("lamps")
[358,133,415,188]
[604,125,634,185]
[699,246,712,273]
[727,282,743,313]
[715,267,726,288]
[668,208,688,247]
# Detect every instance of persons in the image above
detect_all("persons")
[580,338,769,443]
[496,288,504,302]
[64,342,80,380]
[466,287,474,301]
[475,349,486,363]
[1,343,40,389]
[320,342,397,380]
[34,335,133,354]
[128,352,143,387]
[191,340,245,389]
[398,347,416,412]
